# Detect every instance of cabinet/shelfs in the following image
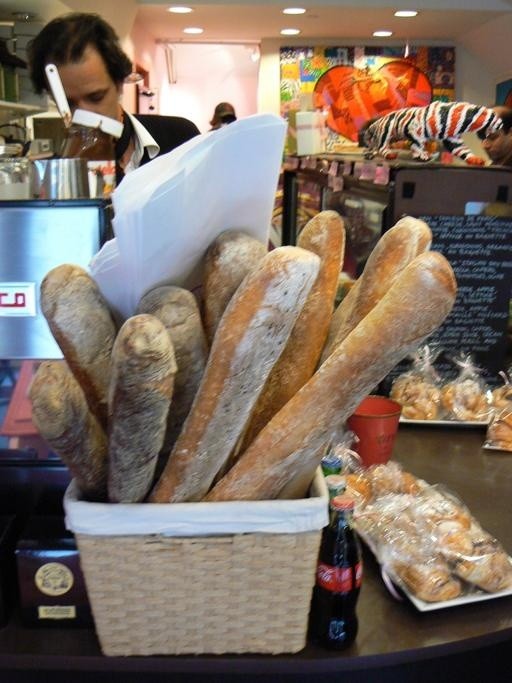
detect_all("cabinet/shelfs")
[268,153,512,401]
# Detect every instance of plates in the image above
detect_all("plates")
[483,433,512,451]
[399,408,495,424]
[355,478,512,612]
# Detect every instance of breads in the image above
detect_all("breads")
[391,368,441,420]
[27,364,107,501]
[345,466,459,602]
[151,247,321,503]
[200,230,269,356]
[205,250,455,503]
[241,209,345,455]
[108,314,177,505]
[443,379,490,421]
[374,459,512,592]
[37,265,115,423]
[486,383,512,450]
[138,285,204,448]
[325,217,432,362]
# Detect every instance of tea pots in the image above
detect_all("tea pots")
[0,123,32,157]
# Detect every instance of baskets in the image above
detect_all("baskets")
[54,465,332,657]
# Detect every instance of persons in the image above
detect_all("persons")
[26,13,202,189]
[477,106,512,166]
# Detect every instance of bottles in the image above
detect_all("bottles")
[320,456,342,486]
[316,497,363,650]
[321,474,347,527]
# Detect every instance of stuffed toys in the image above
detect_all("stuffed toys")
[363,99,503,166]
